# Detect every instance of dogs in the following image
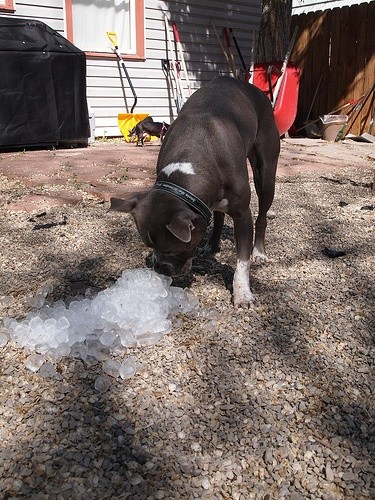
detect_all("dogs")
[135,122,171,146]
[107,77,280,311]
[128,116,153,142]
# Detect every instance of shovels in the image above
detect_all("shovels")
[107,32,158,142]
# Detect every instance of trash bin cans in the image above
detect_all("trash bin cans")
[319,115,348,141]
